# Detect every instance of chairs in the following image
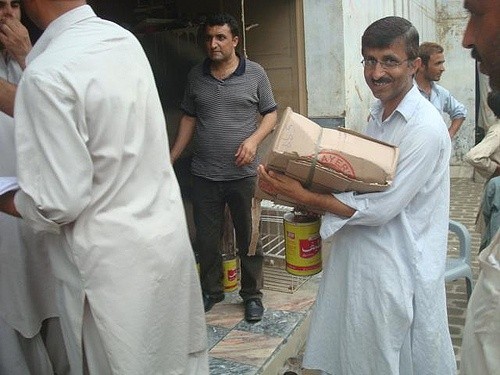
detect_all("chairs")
[444,219,474,306]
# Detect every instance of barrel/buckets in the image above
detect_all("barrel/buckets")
[220,253,239,291]
[194,251,201,276]
[283,212,322,277]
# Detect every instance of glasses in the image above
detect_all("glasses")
[361,57,415,69]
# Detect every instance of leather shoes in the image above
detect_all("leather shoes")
[245,297,265,321]
[203,294,225,312]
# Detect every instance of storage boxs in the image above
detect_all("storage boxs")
[255,107,400,209]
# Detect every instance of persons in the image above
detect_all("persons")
[254,15,459,375]
[415,0,500,375]
[170,11,278,321]
[0,1,211,375]
